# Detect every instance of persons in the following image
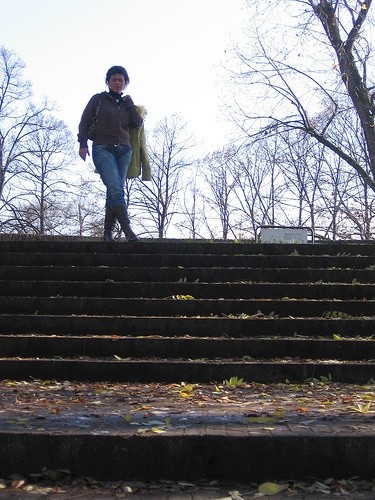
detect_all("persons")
[77,66,142,241]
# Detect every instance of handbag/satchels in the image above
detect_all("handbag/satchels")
[86,93,102,142]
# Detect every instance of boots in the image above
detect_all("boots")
[113,203,138,242]
[102,204,117,243]
